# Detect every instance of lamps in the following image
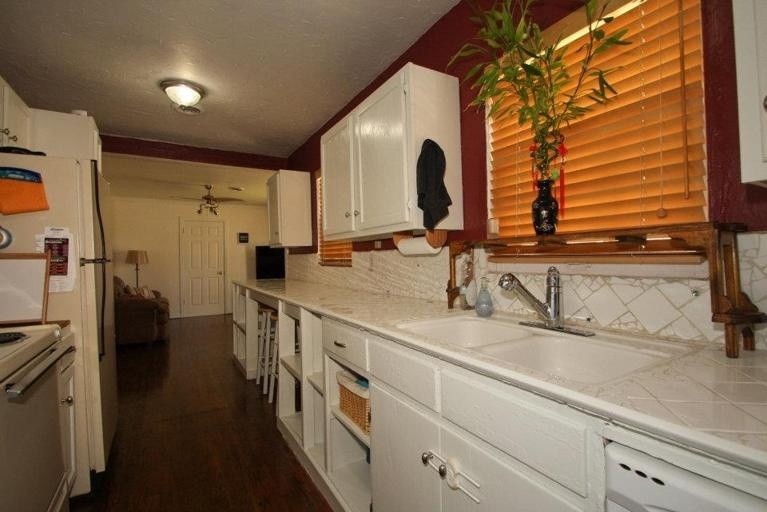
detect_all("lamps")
[157,77,206,106]
[124,248,148,288]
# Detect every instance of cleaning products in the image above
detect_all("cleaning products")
[474,276,495,318]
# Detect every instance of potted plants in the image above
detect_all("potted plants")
[441,0,633,232]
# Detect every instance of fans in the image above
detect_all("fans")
[171,183,245,217]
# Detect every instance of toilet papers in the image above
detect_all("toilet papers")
[395,236,442,257]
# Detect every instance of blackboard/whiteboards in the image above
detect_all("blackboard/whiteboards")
[0,253,50,324]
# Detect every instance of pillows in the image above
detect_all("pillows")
[122,284,157,299]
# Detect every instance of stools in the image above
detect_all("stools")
[263,313,300,403]
[252,307,275,395]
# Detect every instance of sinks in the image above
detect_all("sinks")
[397,313,534,351]
[473,332,700,391]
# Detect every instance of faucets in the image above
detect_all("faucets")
[497,265,563,326]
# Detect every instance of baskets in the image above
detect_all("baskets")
[335,368,370,436]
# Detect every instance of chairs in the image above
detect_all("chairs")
[111,271,172,345]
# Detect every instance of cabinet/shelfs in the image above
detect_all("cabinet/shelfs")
[316,58,463,246]
[24,104,103,174]
[235,286,266,382]
[230,280,237,367]
[0,76,31,150]
[264,167,313,252]
[53,330,83,496]
